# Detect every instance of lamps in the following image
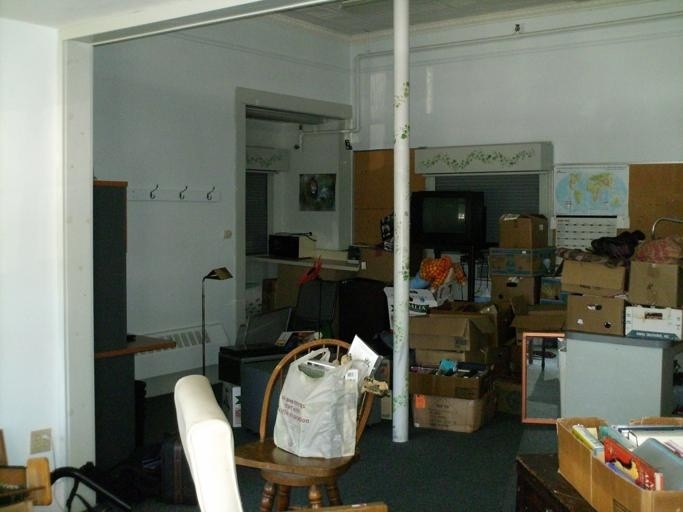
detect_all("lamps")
[201,267,233,376]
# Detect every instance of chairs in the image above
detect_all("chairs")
[1,339,390,512]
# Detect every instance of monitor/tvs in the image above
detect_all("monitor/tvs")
[412,189,486,243]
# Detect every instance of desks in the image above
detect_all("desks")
[249,256,358,278]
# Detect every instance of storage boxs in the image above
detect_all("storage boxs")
[380,216,683,511]
